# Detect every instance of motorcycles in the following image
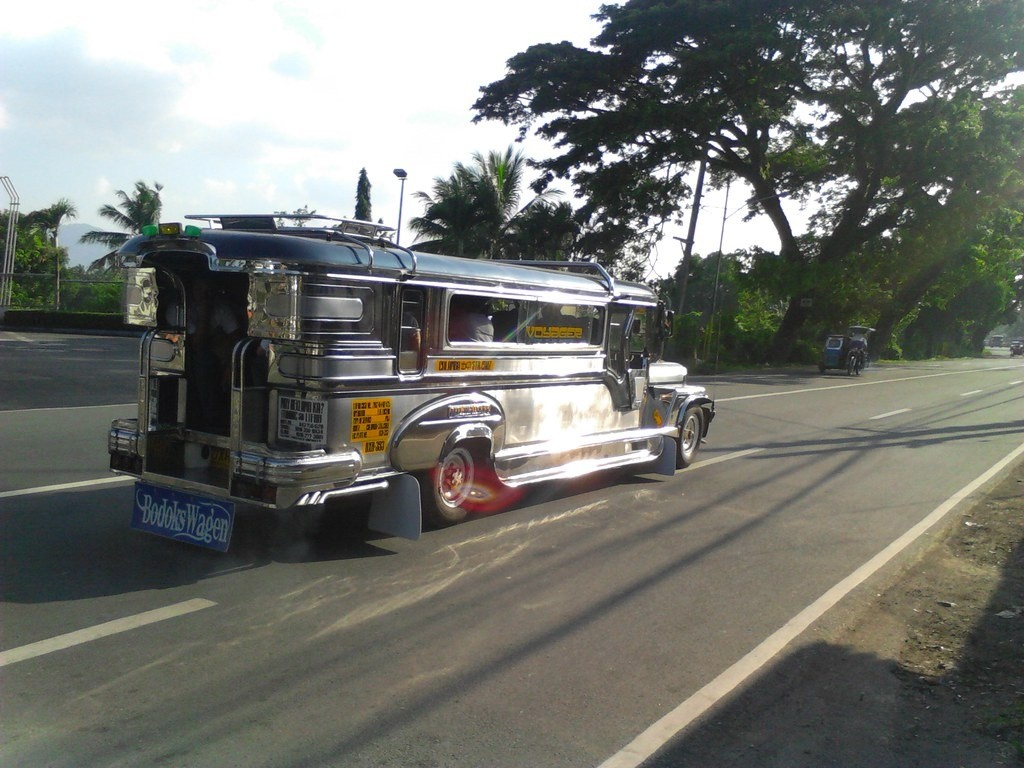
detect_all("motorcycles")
[818,325,874,376]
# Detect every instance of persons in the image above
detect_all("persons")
[852,333,866,349]
[401,298,579,352]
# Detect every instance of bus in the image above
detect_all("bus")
[108,214,716,552]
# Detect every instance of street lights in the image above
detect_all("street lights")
[394,168,407,245]
[705,190,793,360]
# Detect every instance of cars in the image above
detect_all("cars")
[1010,340,1024,357]
[991,336,1003,347]
